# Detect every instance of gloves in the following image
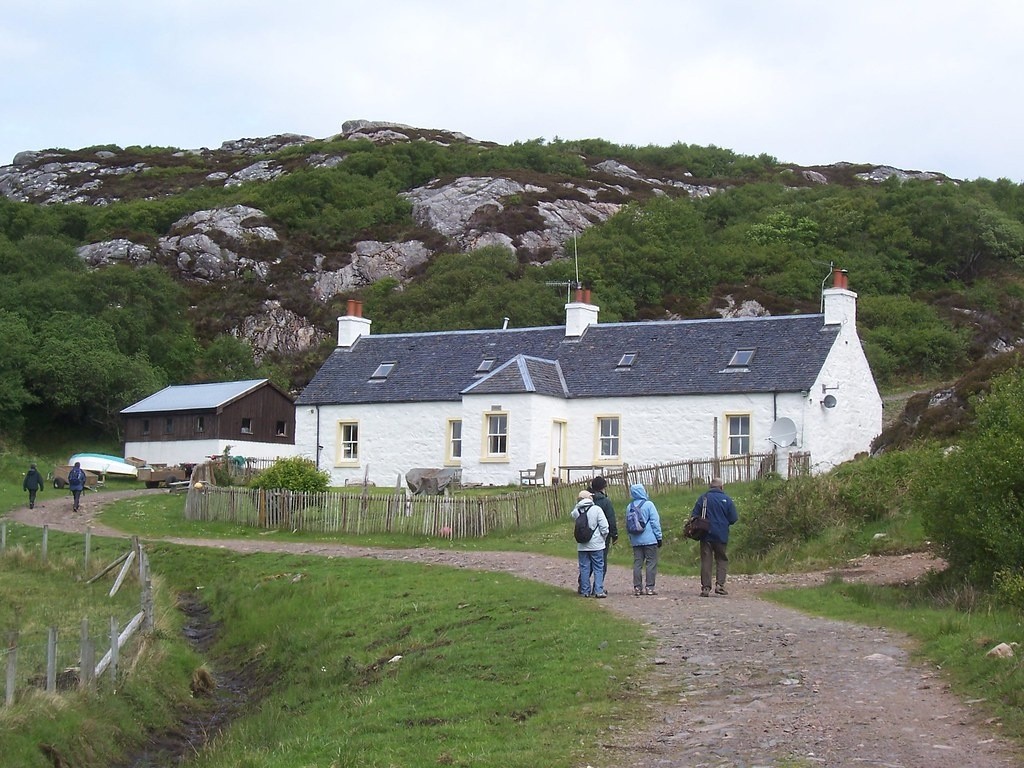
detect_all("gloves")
[657,539,662,548]
[612,534,618,545]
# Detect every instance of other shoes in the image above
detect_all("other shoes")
[595,592,607,597]
[74,507,77,512]
[715,586,728,595]
[700,587,711,597]
[645,587,658,595]
[29,503,34,509]
[634,587,644,595]
[581,592,591,597]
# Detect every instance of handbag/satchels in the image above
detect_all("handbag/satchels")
[684,517,709,541]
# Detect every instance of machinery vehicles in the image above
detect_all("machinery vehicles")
[136,462,199,489]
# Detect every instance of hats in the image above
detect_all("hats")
[711,478,721,487]
[577,490,595,501]
[31,463,36,467]
[592,476,607,490]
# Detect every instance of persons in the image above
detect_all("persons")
[625,484,662,596]
[690,478,737,597]
[23,463,44,509]
[68,462,86,512]
[570,489,609,599]
[577,476,618,594]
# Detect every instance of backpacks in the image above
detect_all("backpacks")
[69,467,82,485]
[574,505,599,543]
[625,499,651,534]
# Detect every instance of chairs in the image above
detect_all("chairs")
[439,469,462,495]
[606,463,629,492]
[519,462,546,490]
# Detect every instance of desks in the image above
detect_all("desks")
[558,465,604,484]
